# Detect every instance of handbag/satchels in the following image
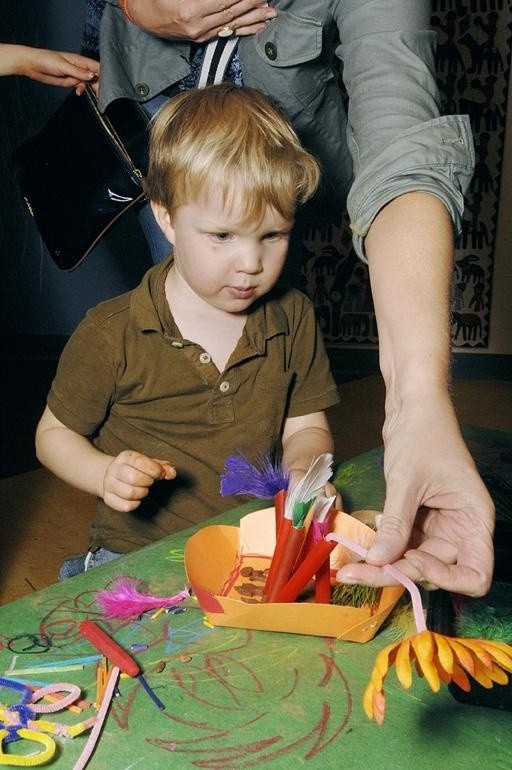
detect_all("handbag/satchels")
[8,37,236,274]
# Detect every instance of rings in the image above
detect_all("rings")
[215,25,233,38]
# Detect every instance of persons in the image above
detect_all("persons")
[3,37,104,97]
[94,0,495,601]
[36,86,342,580]
